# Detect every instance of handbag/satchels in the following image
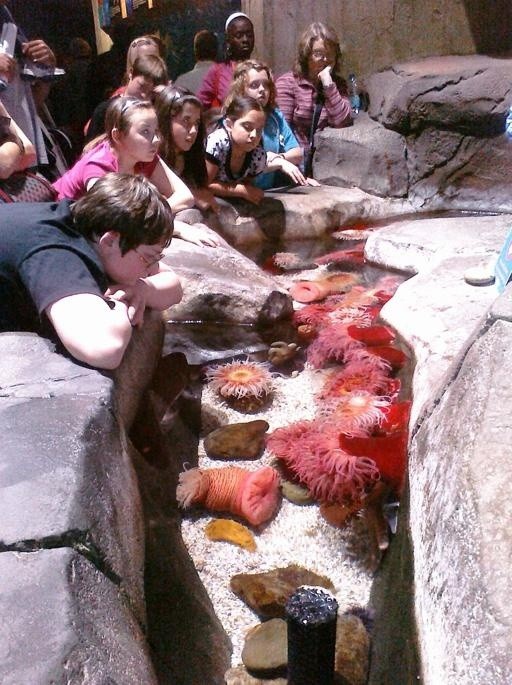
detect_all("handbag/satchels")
[296,145,317,177]
[0,170,59,204]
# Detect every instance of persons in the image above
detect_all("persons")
[0,0,354,372]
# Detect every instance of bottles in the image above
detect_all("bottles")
[347,72,361,110]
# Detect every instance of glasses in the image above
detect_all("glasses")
[133,248,165,269]
[309,51,337,61]
